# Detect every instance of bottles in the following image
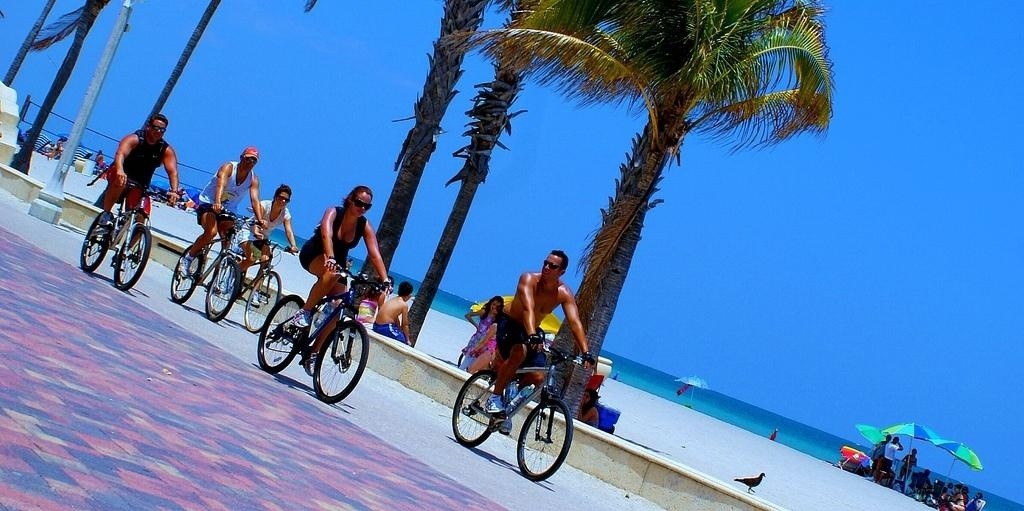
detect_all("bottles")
[508,382,535,408]
[313,299,336,329]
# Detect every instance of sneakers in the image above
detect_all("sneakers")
[484,393,505,413]
[293,308,311,328]
[251,291,260,307]
[97,212,113,227]
[120,260,126,272]
[499,418,512,432]
[304,353,317,376]
[207,282,221,294]
[219,277,235,294]
[179,255,192,278]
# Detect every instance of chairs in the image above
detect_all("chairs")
[835,443,987,510]
[19,126,93,167]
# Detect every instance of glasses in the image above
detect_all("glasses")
[279,196,290,203]
[354,200,372,209]
[247,158,256,165]
[543,260,558,269]
[152,126,166,133]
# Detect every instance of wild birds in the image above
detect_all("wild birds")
[733,472,767,494]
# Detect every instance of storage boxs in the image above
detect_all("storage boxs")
[595,399,622,432]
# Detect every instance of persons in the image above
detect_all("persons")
[833,419,993,511]
[355,277,396,325]
[578,389,603,430]
[468,300,501,378]
[93,110,181,270]
[177,146,267,292]
[483,250,594,436]
[372,280,415,347]
[463,296,503,373]
[770,427,779,441]
[343,257,354,271]
[290,186,393,376]
[42,132,213,213]
[226,183,298,307]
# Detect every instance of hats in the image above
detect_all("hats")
[244,147,259,161]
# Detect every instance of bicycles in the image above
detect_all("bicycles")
[79,176,182,292]
[449,331,590,487]
[208,234,300,335]
[254,266,394,408]
[165,211,268,325]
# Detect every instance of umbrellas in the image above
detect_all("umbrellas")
[467,295,565,334]
[675,375,709,409]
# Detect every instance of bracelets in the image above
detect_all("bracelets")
[169,188,178,193]
